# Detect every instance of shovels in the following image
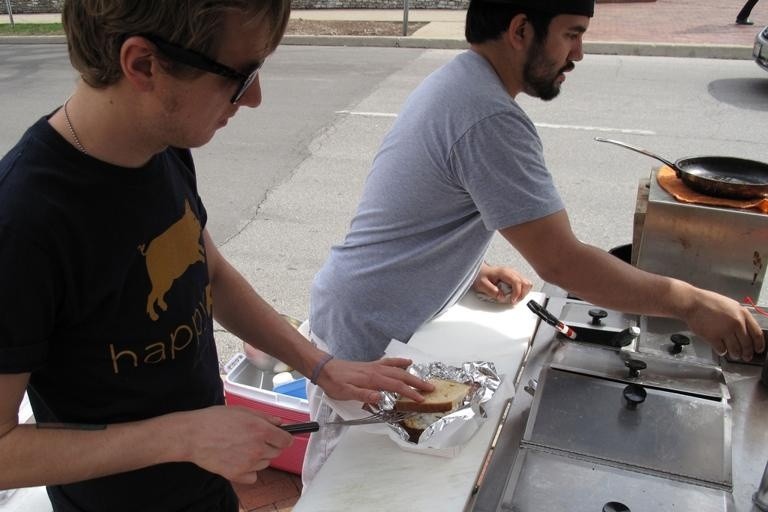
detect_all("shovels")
[277,410,409,433]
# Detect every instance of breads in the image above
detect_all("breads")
[392,378,471,413]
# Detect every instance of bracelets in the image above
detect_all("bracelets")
[311,354,334,386]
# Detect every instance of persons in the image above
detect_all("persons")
[0,0,435,511]
[300,0,766,493]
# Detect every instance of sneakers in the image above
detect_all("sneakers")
[736,20,753,25]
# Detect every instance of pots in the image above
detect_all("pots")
[595,135,768,198]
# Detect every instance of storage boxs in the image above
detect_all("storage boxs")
[220,350,310,477]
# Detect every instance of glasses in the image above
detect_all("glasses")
[159,38,262,105]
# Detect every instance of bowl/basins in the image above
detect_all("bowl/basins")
[244,313,304,374]
